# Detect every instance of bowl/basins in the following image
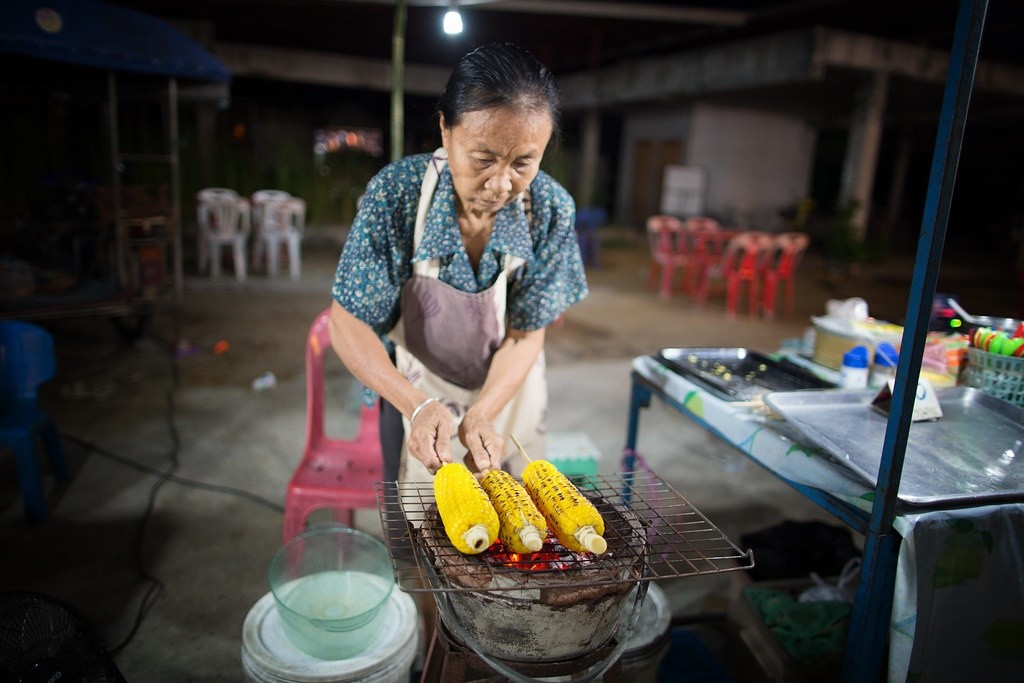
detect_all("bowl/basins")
[268,516,398,663]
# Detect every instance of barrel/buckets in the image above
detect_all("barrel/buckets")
[607,577,672,682]
[238,570,420,683]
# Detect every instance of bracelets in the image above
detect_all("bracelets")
[408,397,440,425]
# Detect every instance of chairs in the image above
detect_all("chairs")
[279,302,397,580]
[192,185,308,284]
[643,212,806,326]
[0,318,68,515]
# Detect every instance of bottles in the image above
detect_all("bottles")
[836,345,869,391]
[872,344,900,387]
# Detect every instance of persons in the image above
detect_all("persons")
[326,36,591,532]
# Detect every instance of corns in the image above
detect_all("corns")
[433,459,608,556]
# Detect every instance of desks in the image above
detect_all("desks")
[618,333,1022,682]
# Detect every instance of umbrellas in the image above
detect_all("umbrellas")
[0,1,229,84]
